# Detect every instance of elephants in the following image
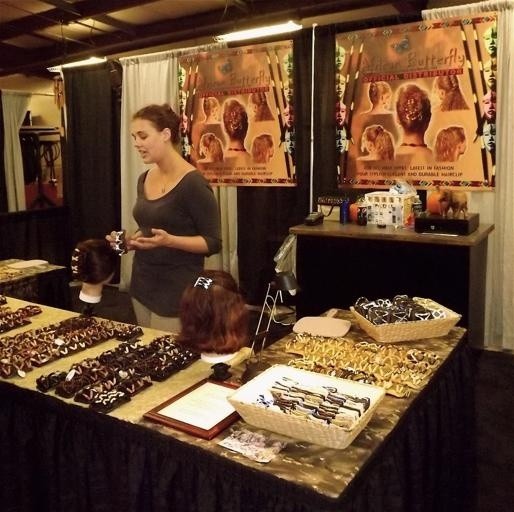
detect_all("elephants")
[438,189,468,219]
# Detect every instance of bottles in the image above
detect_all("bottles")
[357,193,367,226]
[411,195,422,229]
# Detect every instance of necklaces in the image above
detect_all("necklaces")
[228,147,246,151]
[401,142,426,148]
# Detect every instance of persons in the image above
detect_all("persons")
[70,236,119,302]
[202,96,222,125]
[357,125,395,161]
[178,65,191,157]
[434,74,470,111]
[434,127,466,162]
[249,92,275,121]
[480,27,496,152]
[393,83,433,154]
[196,133,223,163]
[363,81,394,115]
[334,46,349,153]
[176,269,251,364]
[221,99,253,160]
[282,54,296,154]
[251,134,275,163]
[102,102,224,337]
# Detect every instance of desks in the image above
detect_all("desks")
[0,257,481,512]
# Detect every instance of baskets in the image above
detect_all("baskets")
[365,191,416,226]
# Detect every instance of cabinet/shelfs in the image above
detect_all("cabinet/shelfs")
[289,221,495,349]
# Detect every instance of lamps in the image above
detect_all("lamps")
[210,1,303,42]
[47,18,107,73]
